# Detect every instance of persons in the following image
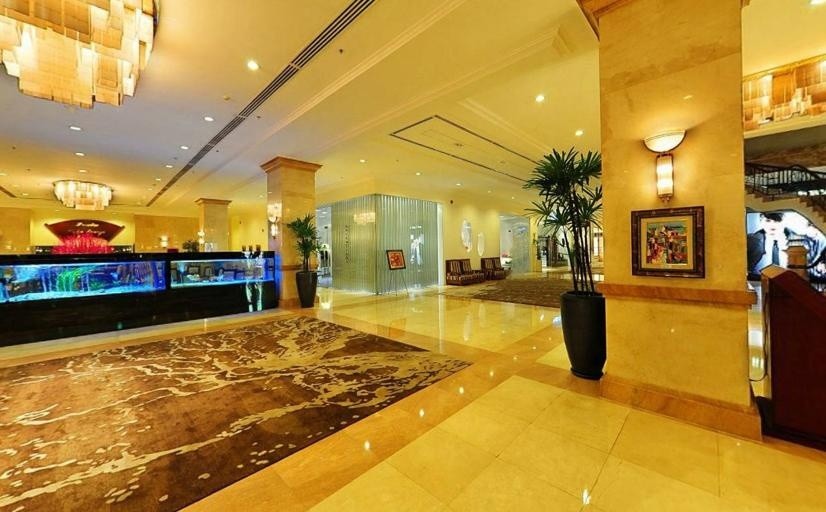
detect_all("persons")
[748,212,809,280]
[116,264,135,280]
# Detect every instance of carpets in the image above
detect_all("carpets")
[472,275,601,310]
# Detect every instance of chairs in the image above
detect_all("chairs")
[446,257,507,285]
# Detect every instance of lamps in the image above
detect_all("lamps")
[641,130,686,200]
[0,0,161,213]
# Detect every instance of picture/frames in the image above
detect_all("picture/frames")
[630,206,704,278]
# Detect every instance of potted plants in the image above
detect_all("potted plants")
[286,213,328,309]
[520,143,605,380]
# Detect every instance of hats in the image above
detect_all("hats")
[760,211,784,222]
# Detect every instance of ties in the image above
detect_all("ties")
[772,240,780,267]
